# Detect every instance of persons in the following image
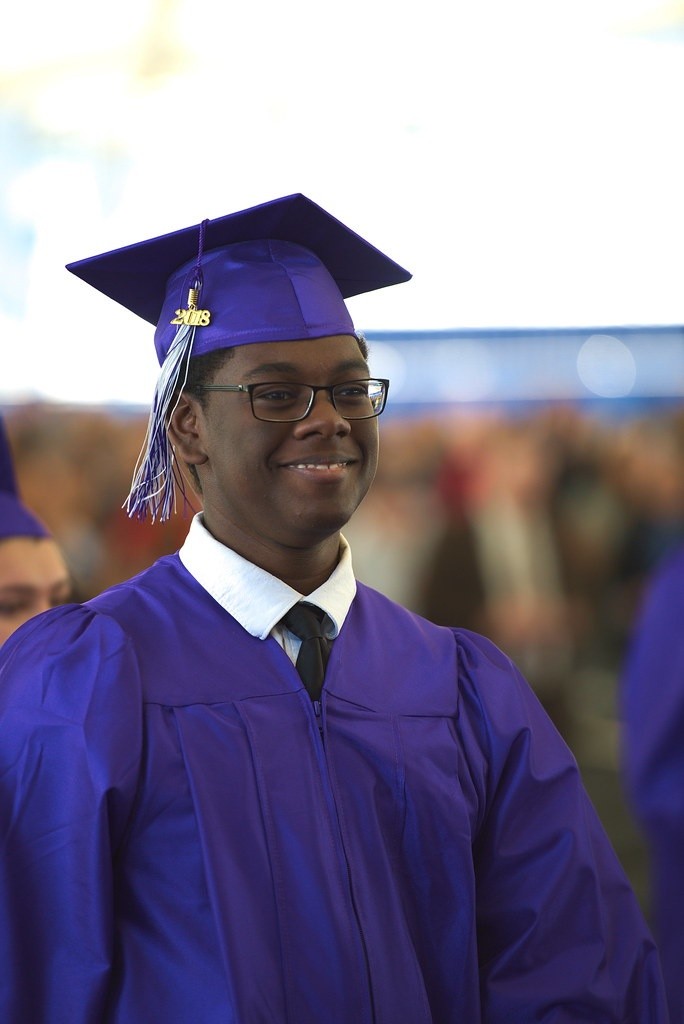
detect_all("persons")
[0,193,684,1024]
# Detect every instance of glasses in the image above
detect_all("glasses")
[191,375,390,423]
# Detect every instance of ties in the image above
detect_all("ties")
[278,600,331,702]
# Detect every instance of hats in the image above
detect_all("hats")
[0,418,48,538]
[65,192,412,528]
